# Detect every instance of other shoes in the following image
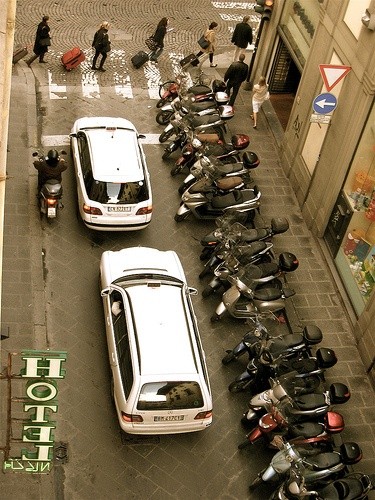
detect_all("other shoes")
[39,60,48,63]
[249,114,254,120]
[26,61,32,68]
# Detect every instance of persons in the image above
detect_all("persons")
[250,75,268,128]
[224,53,248,107]
[196,22,218,67]
[112,301,124,316]
[33,149,68,193]
[91,22,112,72]
[231,15,253,62]
[26,16,52,67]
[150,17,169,63]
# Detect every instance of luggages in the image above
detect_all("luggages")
[180,51,210,70]
[12,43,33,65]
[61,47,93,72]
[131,50,154,69]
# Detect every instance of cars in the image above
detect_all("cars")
[99,246,214,436]
[68,117,153,232]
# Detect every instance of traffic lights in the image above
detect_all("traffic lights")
[260,0,274,22]
[253,0,265,14]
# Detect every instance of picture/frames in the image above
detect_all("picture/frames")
[353,236,373,263]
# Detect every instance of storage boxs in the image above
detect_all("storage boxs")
[214,92,231,105]
[212,79,227,94]
[217,105,235,121]
[230,134,250,151]
[242,151,260,170]
[329,383,351,405]
[302,325,323,345]
[339,441,363,465]
[41,179,63,199]
[278,251,299,272]
[270,216,289,234]
[315,348,338,369]
[324,411,345,434]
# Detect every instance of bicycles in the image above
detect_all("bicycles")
[159,70,212,99]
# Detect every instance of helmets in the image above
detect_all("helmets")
[48,150,58,158]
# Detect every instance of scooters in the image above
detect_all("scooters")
[222,312,324,365]
[238,398,346,449]
[156,78,228,125]
[240,368,352,426]
[248,435,363,491]
[228,331,338,394]
[269,466,375,500]
[158,90,230,143]
[210,262,297,326]
[161,105,290,261]
[202,247,299,299]
[32,150,68,226]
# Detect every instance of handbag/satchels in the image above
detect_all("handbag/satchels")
[198,31,215,50]
[145,34,160,51]
[264,84,271,101]
[37,37,51,46]
